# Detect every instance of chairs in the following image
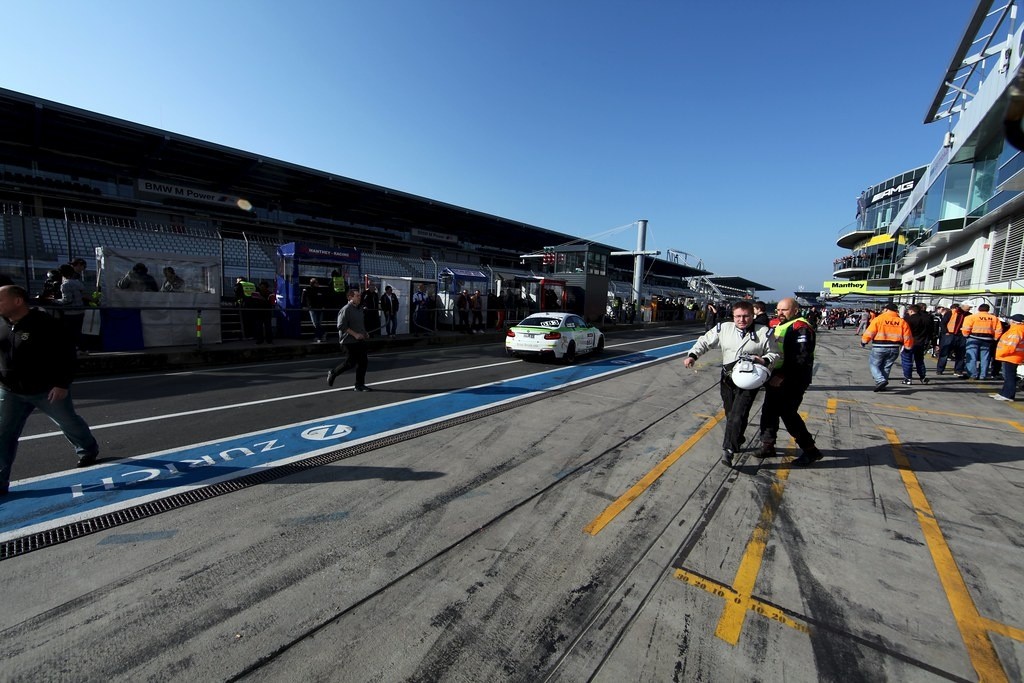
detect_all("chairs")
[0,215,491,277]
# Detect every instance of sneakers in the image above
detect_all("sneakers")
[327,369,335,386]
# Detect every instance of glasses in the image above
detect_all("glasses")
[731,313,754,319]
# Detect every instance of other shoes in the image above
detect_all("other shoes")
[479,329,485,333]
[472,328,476,334]
[353,383,370,392]
[900,378,912,385]
[953,371,963,376]
[921,376,930,384]
[751,445,775,457]
[989,393,1014,400]
[77,445,99,467]
[722,449,733,468]
[935,371,943,375]
[792,446,823,467]
[874,380,888,393]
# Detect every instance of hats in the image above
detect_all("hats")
[1011,314,1024,322]
[67,257,87,270]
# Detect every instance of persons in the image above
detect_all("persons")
[685,301,782,468]
[754,301,770,329]
[362,284,379,337]
[989,314,1024,402]
[861,304,914,392]
[496,290,505,332]
[118,262,159,292]
[234,276,259,340]
[457,289,474,335]
[962,304,1002,380]
[471,290,485,333]
[413,283,429,337]
[0,285,100,503]
[900,303,928,385]
[55,258,87,307]
[327,289,372,392]
[255,280,277,344]
[160,267,185,293]
[797,303,972,377]
[505,289,514,320]
[381,286,399,338]
[300,277,328,342]
[751,296,825,467]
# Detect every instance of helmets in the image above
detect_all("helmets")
[731,358,771,389]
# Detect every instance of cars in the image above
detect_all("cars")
[505,312,606,365]
